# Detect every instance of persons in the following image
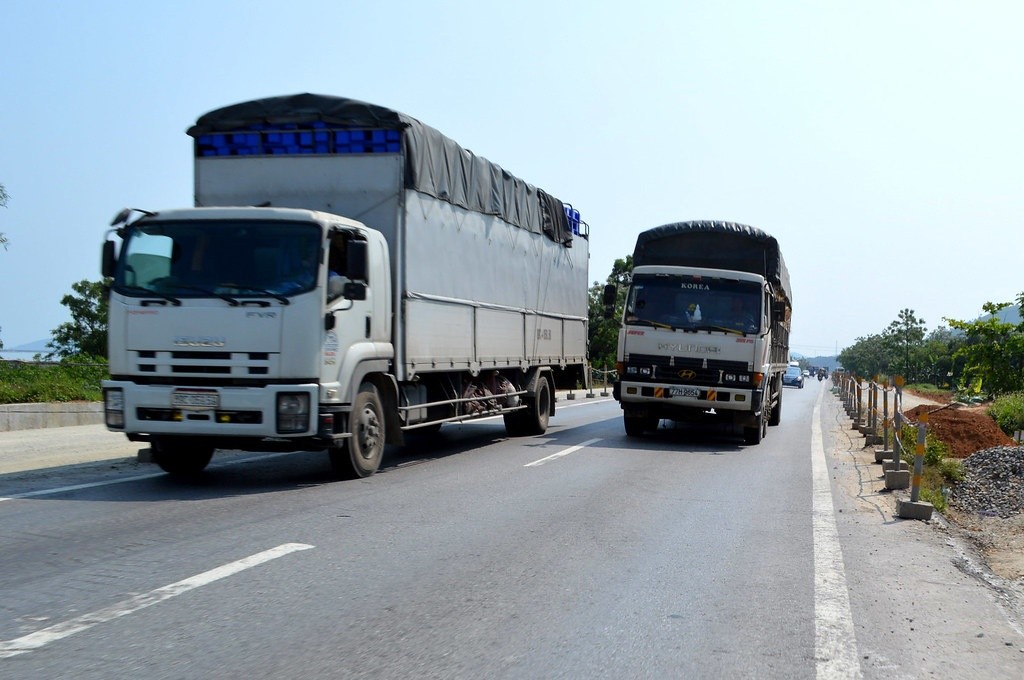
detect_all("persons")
[714,294,756,334]
[817,369,824,376]
[296,252,339,298]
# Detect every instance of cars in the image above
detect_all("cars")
[802,370,810,378]
[782,367,804,388]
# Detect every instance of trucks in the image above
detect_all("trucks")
[603,220,792,445]
[100,93,589,479]
[789,362,799,367]
[807,366,828,376]
